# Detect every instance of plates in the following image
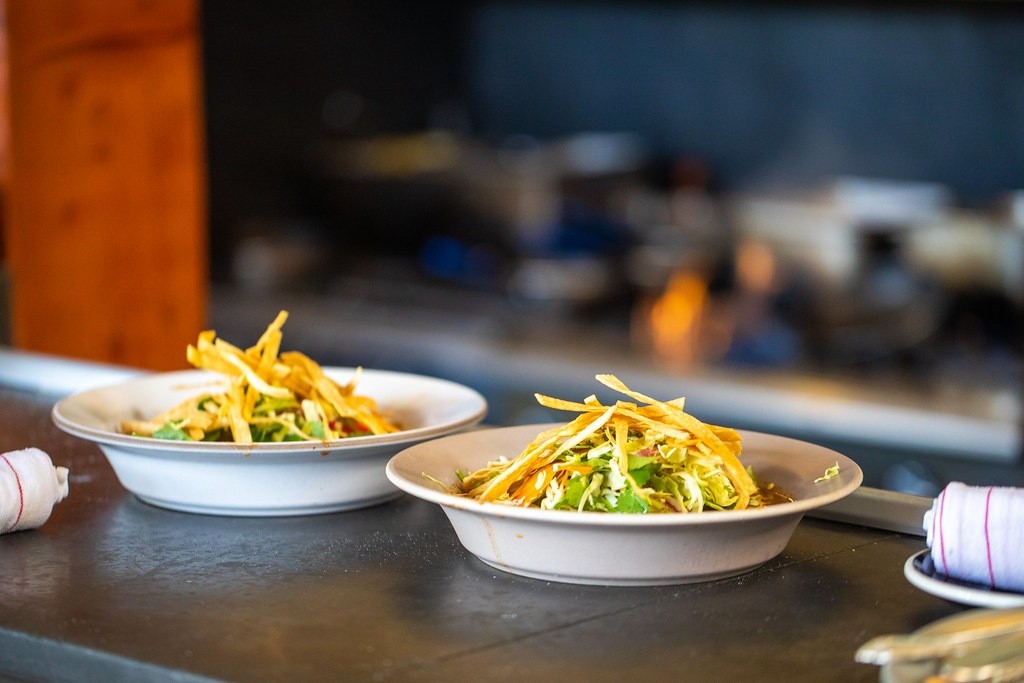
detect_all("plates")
[901,548,1024,609]
[383,420,864,587]
[878,608,1024,683]
[52,367,489,516]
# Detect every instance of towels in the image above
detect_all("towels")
[1,446,70,536]
[922,479,1021,592]
